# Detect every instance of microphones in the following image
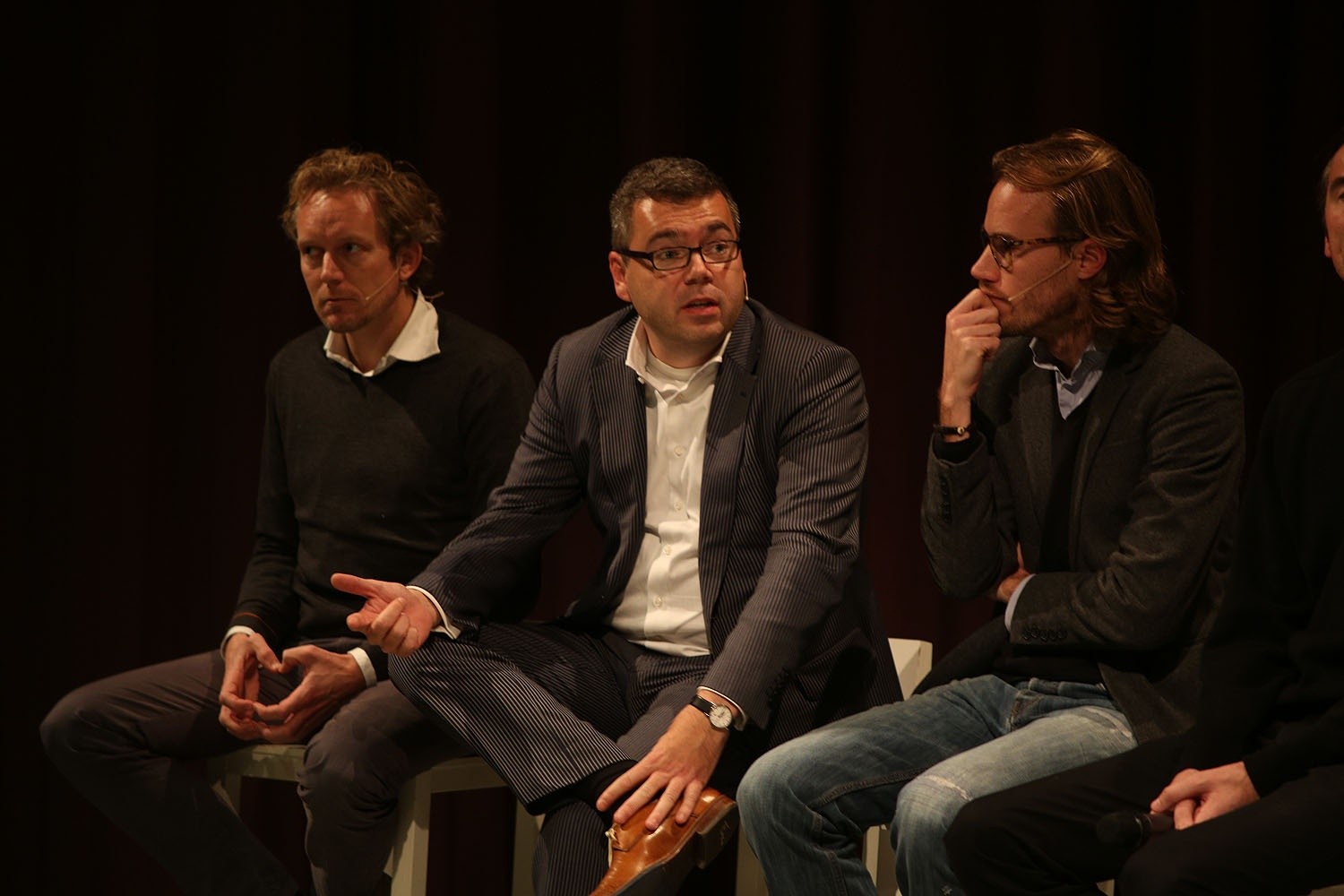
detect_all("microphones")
[365,251,402,301]
[1007,238,1081,302]
[743,277,749,301]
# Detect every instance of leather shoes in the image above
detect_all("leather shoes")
[588,786,740,896]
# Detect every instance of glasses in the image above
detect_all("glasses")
[613,240,742,271]
[983,228,1092,269]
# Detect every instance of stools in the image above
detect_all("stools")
[511,639,934,896]
[225,738,547,895]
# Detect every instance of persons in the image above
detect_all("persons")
[331,159,905,895]
[941,132,1342,894]
[736,133,1245,894]
[37,149,535,896]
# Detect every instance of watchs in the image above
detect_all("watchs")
[689,695,738,736]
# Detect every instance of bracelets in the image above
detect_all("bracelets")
[933,419,977,435]
